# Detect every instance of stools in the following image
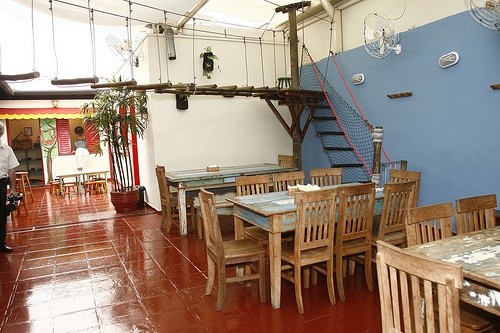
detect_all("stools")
[15,172,35,215]
[193,196,234,239]
[49,177,107,198]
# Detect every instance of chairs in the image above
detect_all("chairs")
[155,155,500,333]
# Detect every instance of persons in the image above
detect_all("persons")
[0,122,20,252]
[74,126,90,171]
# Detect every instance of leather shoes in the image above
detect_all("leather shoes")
[2,247,13,252]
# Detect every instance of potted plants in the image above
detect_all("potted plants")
[81,75,149,212]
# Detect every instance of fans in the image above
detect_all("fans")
[363,11,401,59]
[465,0,500,31]
[105,35,139,67]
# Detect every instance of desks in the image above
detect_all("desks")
[84,169,108,189]
[403,228,500,314]
[165,163,298,237]
[226,183,386,310]
[56,169,84,194]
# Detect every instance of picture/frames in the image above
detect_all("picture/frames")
[24,127,32,136]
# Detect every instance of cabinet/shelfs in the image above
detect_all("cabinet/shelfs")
[11,142,43,180]
